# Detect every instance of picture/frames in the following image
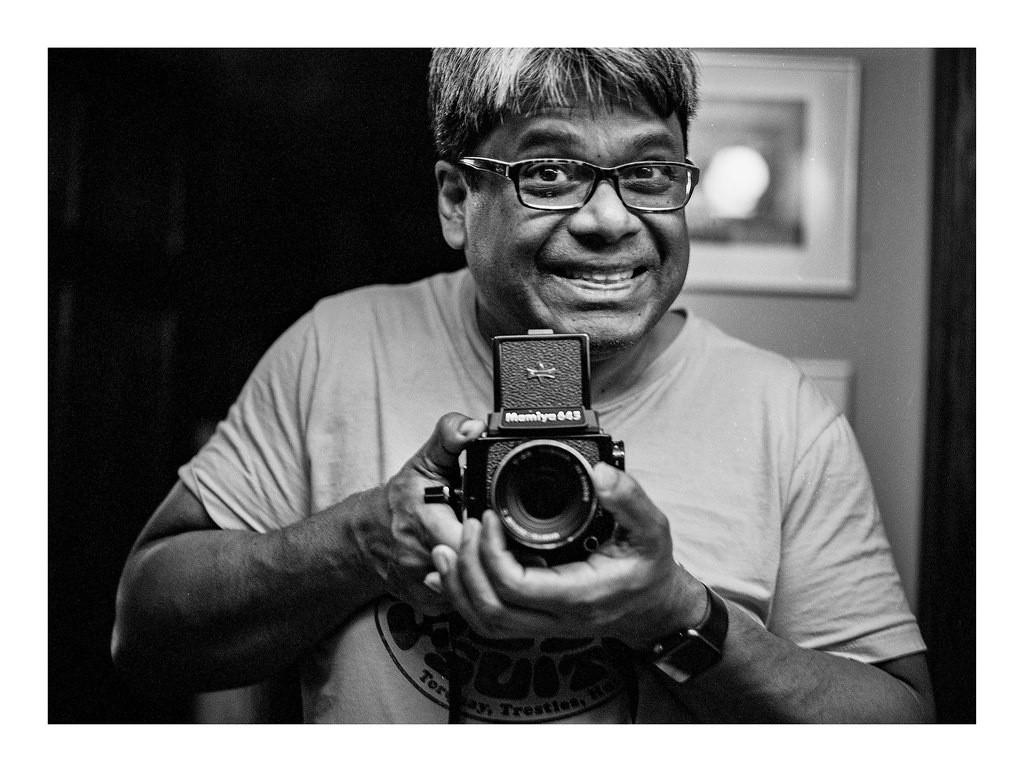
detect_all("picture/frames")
[669,50,865,303]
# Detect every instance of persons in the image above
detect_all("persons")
[108,47,934,723]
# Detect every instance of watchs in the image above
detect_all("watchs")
[651,584,730,686]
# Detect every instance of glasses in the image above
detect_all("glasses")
[458,158,701,211]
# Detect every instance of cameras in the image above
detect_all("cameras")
[426,330,625,569]
